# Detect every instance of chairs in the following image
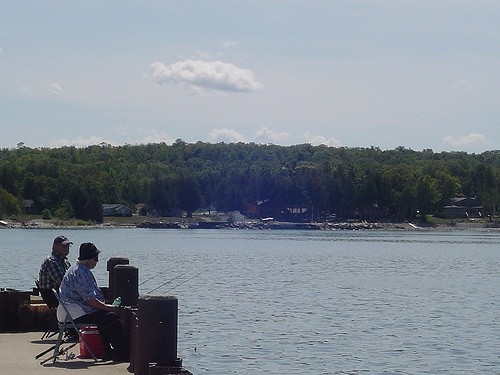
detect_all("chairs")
[40,288,101,367]
[32,281,74,364]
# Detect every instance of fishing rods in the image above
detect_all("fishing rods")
[33,267,210,365]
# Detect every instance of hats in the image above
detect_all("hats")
[77,242,102,259]
[54,235,73,245]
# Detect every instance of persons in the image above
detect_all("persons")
[38,235,80,344]
[56,242,130,362]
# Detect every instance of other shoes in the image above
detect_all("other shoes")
[101,349,130,364]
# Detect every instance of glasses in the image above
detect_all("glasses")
[90,257,98,261]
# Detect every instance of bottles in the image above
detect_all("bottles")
[112,297,121,308]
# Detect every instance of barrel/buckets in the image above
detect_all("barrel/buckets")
[78,326,105,358]
